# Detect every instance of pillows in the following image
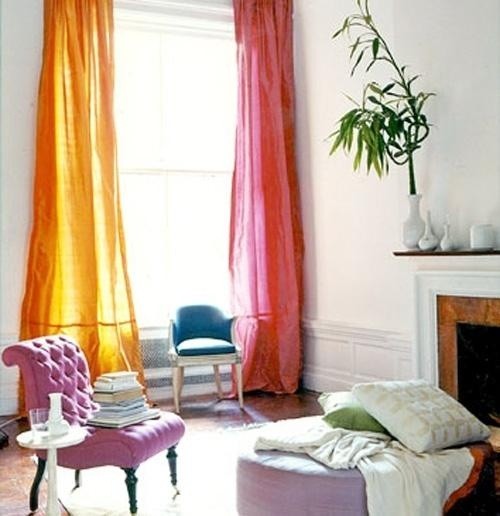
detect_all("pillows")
[316,391,385,434]
[352,379,490,450]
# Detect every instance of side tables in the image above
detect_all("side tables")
[15,427,87,516]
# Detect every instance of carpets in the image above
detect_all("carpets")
[30,412,500,516]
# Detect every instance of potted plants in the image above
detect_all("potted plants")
[326,0,436,248]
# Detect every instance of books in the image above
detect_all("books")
[87,372,161,430]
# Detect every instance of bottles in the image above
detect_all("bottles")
[49,393,72,437]
[419,210,458,251]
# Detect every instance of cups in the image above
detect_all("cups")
[30,408,48,438]
[469,224,495,250]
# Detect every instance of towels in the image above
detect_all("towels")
[300,426,475,516]
[253,415,330,455]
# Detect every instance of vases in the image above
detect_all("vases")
[418,210,438,250]
[439,224,454,250]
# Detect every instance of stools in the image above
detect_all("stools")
[235,440,493,516]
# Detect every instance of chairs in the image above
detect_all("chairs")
[3,333,185,516]
[167,304,244,414]
